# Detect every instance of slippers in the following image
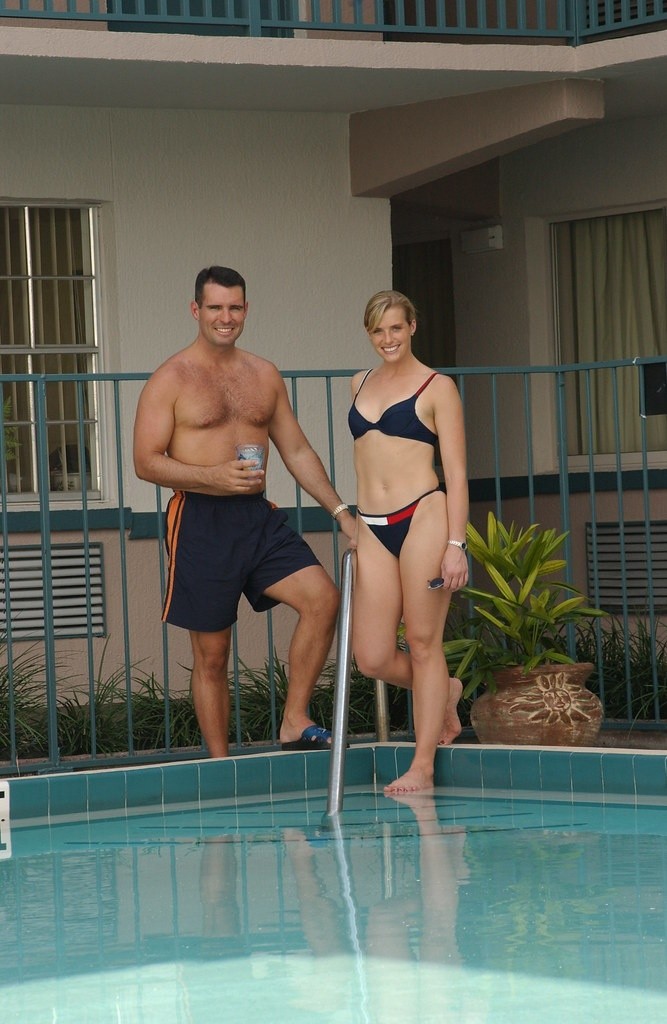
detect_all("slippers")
[281,725,350,750]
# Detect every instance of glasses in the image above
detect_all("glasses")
[428,578,444,590]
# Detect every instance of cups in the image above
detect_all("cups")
[237,445,265,481]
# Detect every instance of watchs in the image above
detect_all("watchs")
[447,540,468,551]
[330,503,349,519]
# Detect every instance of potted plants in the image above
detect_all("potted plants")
[440,510,604,748]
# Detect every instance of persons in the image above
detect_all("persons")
[132,266,359,761]
[345,290,471,793]
[199,785,484,1024]
